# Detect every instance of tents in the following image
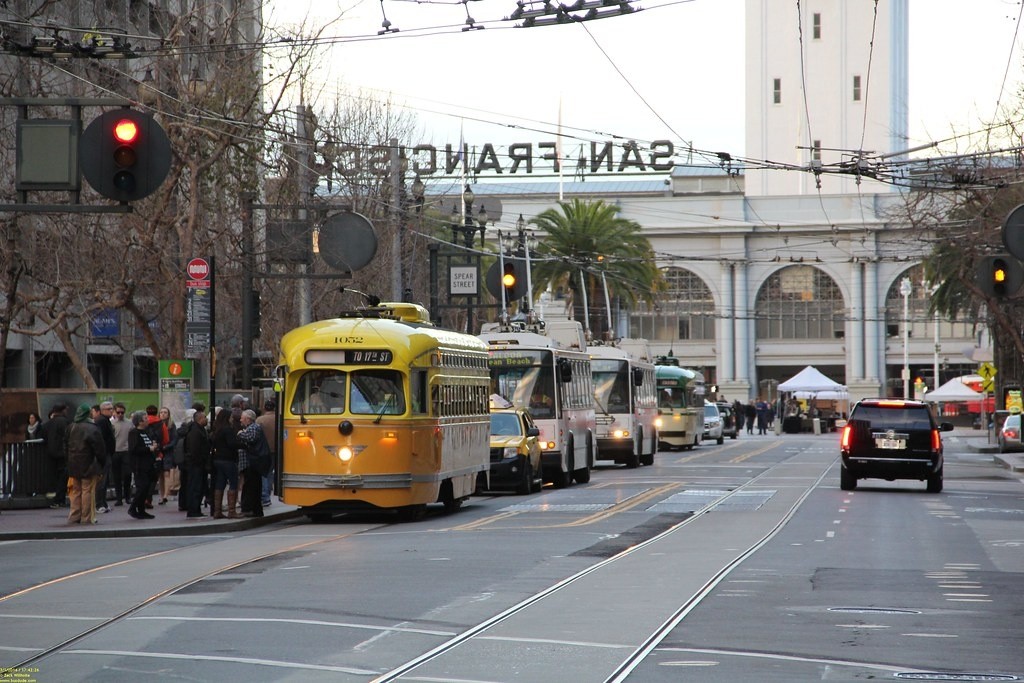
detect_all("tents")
[792,391,850,418]
[777,365,842,421]
[925,380,984,429]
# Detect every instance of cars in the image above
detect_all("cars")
[996,413,1024,453]
[700,398,727,445]
[715,400,740,440]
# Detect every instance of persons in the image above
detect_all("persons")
[745,400,756,434]
[527,393,551,405]
[128,405,177,520]
[732,400,745,430]
[755,398,776,435]
[793,395,803,411]
[178,394,276,518]
[719,395,727,403]
[25,412,40,441]
[36,399,136,525]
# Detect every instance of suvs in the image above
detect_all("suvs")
[833,396,954,494]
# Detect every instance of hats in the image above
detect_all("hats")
[232,394,248,403]
[74,403,90,422]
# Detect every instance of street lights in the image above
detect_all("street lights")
[449,183,489,337]
[379,144,426,301]
[503,212,539,333]
[283,102,337,324]
[135,61,206,364]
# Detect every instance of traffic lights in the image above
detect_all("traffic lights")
[484,255,529,302]
[77,108,172,202]
[976,250,1024,298]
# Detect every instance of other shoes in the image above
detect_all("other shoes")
[50,502,68,508]
[127,499,132,504]
[186,512,208,518]
[95,506,110,513]
[242,511,255,517]
[145,503,154,509]
[113,500,123,506]
[156,497,167,505]
[127,510,142,519]
[137,511,156,519]
[261,501,271,507]
[92,518,97,523]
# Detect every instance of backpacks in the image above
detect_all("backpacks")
[247,431,270,478]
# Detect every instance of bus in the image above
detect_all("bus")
[472,229,598,498]
[651,348,706,453]
[577,265,659,469]
[272,286,494,525]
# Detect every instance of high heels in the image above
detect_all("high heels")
[204,498,212,507]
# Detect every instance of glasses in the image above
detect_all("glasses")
[115,409,124,414]
[102,408,112,411]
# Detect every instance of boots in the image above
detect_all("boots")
[227,489,245,519]
[213,490,227,520]
